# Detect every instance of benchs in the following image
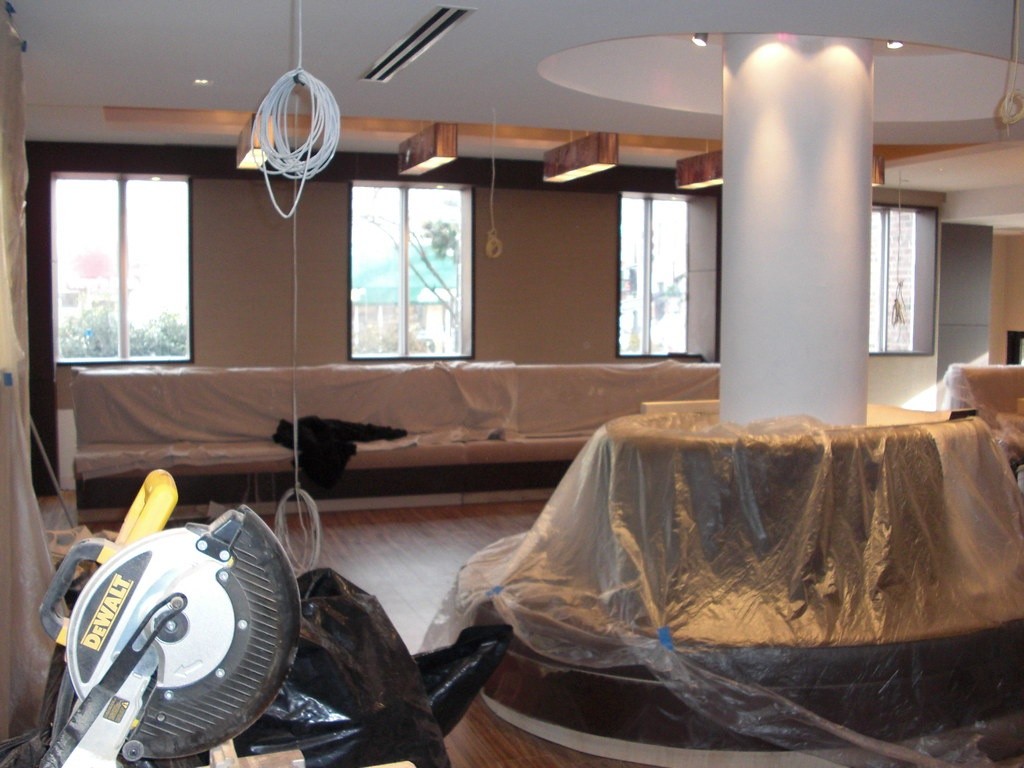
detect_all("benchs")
[74,362,720,523]
[461,405,1024,767]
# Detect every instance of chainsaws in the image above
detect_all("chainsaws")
[35,504,303,768]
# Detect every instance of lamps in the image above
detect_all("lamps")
[886,40,903,48]
[545,130,619,183]
[398,122,458,175]
[691,33,709,46]
[676,150,725,189]
[235,113,274,170]
[871,152,885,187]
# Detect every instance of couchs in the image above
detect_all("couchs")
[949,363,1024,462]
